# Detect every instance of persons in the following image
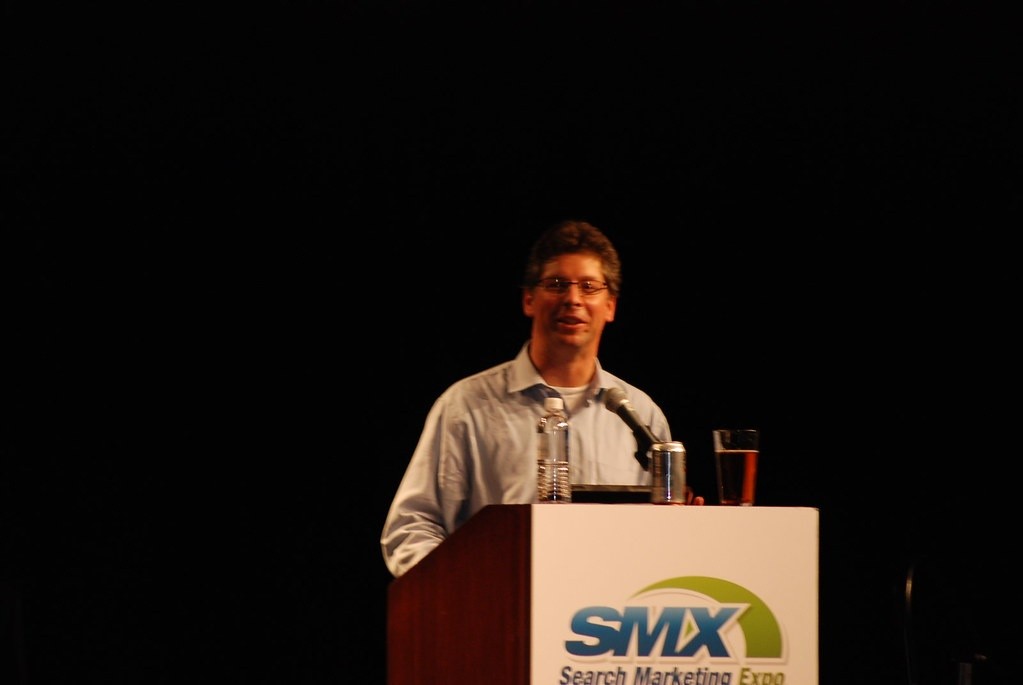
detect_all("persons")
[380,222,703,579]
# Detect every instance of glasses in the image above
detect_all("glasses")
[531,276,610,295]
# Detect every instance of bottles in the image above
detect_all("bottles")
[534,397,574,504]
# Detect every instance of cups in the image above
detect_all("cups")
[712,429,760,507]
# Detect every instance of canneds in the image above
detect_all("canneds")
[649,441,686,505]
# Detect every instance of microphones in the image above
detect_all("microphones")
[605,386,659,446]
